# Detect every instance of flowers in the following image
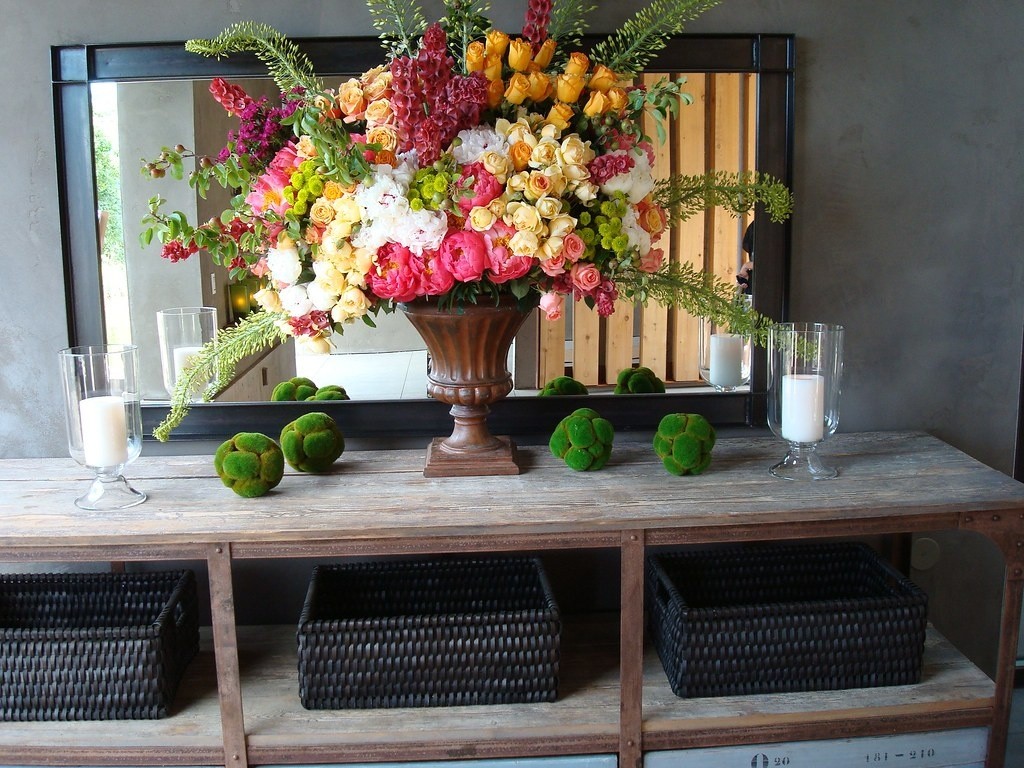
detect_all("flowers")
[136,0,825,446]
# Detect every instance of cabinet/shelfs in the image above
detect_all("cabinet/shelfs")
[3,434,1024,768]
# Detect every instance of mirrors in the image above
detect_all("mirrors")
[45,34,801,436]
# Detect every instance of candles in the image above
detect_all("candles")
[780,374,827,444]
[172,345,211,391]
[708,334,744,388]
[77,396,129,470]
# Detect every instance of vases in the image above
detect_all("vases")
[393,296,546,476]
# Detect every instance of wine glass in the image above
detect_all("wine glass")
[768,323,845,481]
[156,306,219,403]
[698,292,752,391]
[58,342,147,512]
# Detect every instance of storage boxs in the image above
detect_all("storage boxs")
[646,538,935,698]
[292,551,571,710]
[2,566,202,722]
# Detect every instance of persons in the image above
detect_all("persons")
[736,256,754,315]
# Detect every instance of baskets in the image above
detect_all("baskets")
[1,568,200,721]
[298,557,559,712]
[651,537,929,699]
